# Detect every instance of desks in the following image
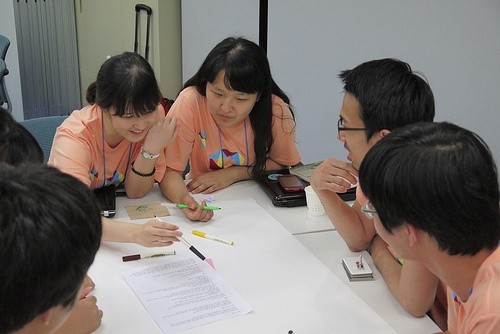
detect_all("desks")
[90,160,444,334]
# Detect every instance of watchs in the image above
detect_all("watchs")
[140,144,161,159]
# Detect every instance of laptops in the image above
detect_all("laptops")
[254,169,356,207]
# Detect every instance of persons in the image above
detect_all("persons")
[0,160,102,334]
[357,120,500,334]
[165,37,302,223]
[45,52,184,249]
[0,106,44,168]
[310,58,449,331]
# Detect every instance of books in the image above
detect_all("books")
[289,164,359,191]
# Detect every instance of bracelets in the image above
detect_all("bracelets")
[131,160,156,177]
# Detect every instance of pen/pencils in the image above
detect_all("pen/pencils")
[191,230,232,246]
[122,249,175,263]
[160,203,222,210]
[153,215,206,261]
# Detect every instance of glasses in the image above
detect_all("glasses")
[337,119,390,140]
[361,202,377,219]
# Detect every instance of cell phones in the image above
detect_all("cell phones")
[277,175,305,193]
